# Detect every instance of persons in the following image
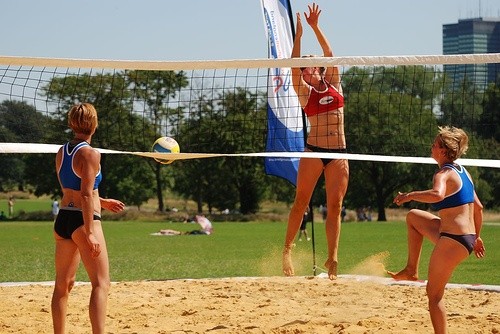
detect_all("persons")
[387,126,485,334]
[321,204,376,222]
[9,195,14,217]
[298,205,310,242]
[52,198,59,222]
[283,3,348,280]
[51,102,125,334]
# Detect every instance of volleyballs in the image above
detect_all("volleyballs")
[151,135,181,166]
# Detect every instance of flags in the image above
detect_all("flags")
[261,0,304,187]
